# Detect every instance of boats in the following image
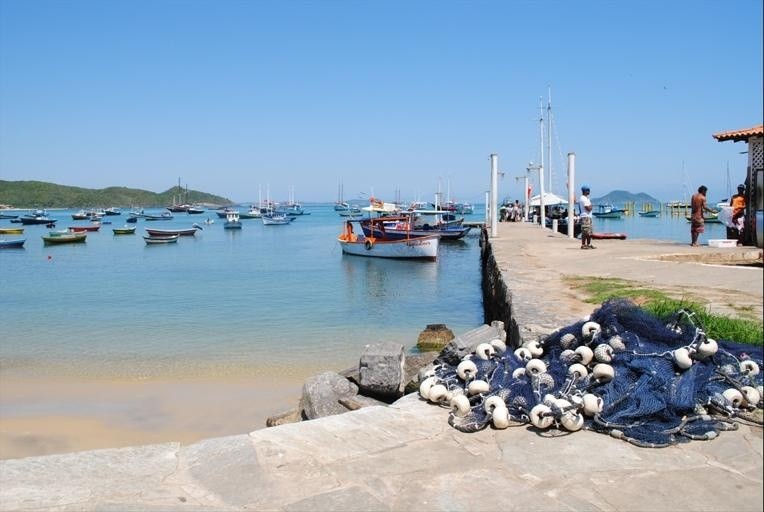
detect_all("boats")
[639,210,661,218]
[665,201,731,223]
[592,204,628,219]
[593,232,627,239]
[334,176,474,262]
[0,175,259,247]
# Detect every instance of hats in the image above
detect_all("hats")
[581,184,590,192]
[737,183,746,191]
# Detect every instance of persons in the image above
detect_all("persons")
[689,186,718,247]
[536,205,569,220]
[500,199,520,221]
[579,186,596,250]
[729,184,746,245]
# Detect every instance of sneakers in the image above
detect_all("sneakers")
[580,245,596,250]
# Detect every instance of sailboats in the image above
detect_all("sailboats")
[249,185,311,226]
[501,86,582,239]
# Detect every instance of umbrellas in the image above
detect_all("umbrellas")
[529,192,568,207]
[522,194,560,206]
